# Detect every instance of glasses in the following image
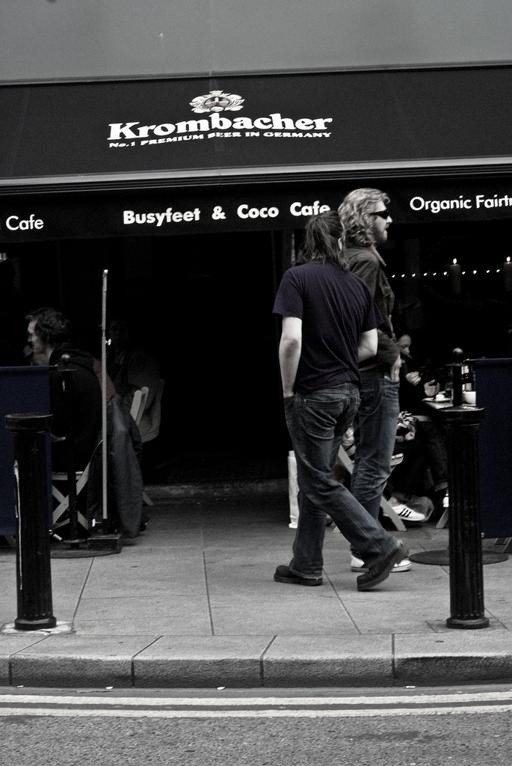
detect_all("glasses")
[368,207,389,219]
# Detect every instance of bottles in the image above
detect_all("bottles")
[461,365,473,391]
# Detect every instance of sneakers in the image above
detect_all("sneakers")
[274,565,322,586]
[351,541,413,591]
[382,504,425,522]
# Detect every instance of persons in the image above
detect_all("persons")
[270,211,411,591]
[337,187,426,574]
[391,330,442,527]
[2,308,144,550]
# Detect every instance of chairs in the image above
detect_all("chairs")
[51,461,96,535]
[128,385,155,506]
[331,444,408,532]
